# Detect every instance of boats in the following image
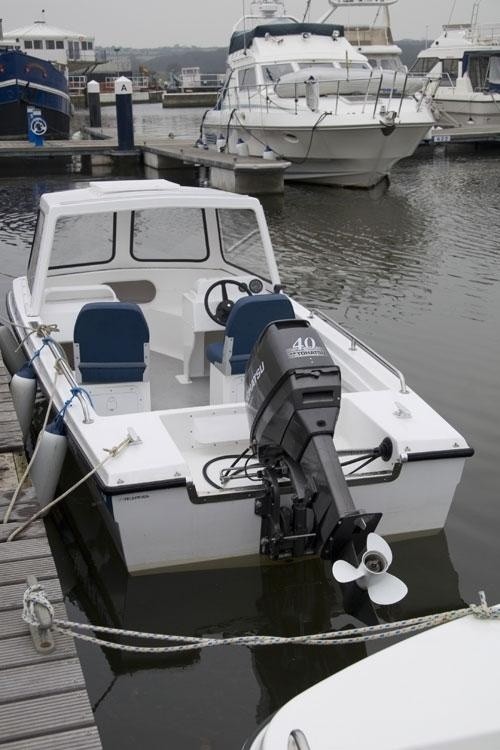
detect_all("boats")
[162,91,223,108]
[0,47,72,140]
[0,177,475,608]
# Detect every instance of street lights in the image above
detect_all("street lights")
[114,45,121,78]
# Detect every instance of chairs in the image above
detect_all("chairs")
[204,293,296,376]
[73,301,151,384]
